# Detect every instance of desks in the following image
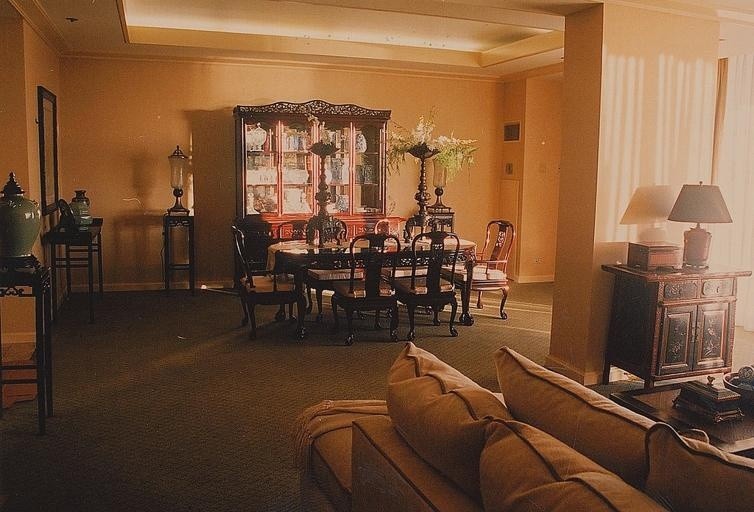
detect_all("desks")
[39,215,104,324]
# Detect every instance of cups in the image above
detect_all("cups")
[356,164,375,184]
[336,195,348,212]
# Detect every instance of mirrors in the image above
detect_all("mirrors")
[34,84,60,219]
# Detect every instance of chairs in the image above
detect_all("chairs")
[226,211,517,347]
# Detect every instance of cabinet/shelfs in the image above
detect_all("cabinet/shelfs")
[230,99,393,291]
[598,261,752,390]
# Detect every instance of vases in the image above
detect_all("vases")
[75,210,93,232]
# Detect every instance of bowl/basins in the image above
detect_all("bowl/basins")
[721,372,753,405]
[288,170,308,184]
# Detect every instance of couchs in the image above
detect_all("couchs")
[281,385,513,511]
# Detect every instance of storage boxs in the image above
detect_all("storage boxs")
[625,241,684,273]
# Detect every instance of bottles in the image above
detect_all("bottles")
[74,204,92,231]
[355,129,367,153]
[251,123,267,151]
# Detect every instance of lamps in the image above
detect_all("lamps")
[618,183,677,245]
[161,143,192,217]
[663,179,735,273]
[423,157,455,215]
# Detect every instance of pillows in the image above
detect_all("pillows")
[469,413,673,511]
[636,419,753,512]
[384,338,518,506]
[486,340,711,496]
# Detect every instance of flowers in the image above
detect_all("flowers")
[379,100,470,189]
